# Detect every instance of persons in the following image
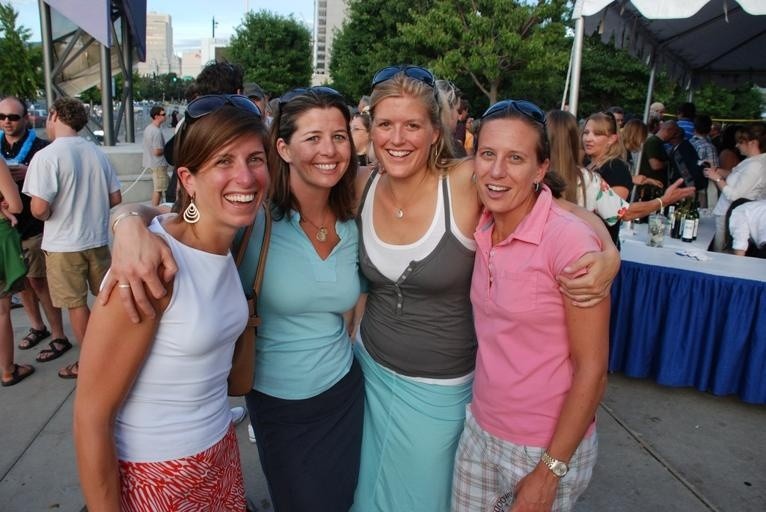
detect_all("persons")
[244,84,276,136]
[608,107,623,130]
[723,198,766,259]
[435,79,468,159]
[349,65,621,511]
[450,100,611,512]
[709,123,725,155]
[22,96,122,378]
[663,103,699,153]
[143,107,172,207]
[99,86,372,512]
[668,126,708,209]
[582,111,632,252]
[647,103,663,137]
[452,101,469,146]
[348,96,373,166]
[0,157,36,386]
[640,121,678,223]
[622,118,664,188]
[689,115,720,209]
[171,106,179,128]
[719,125,747,171]
[0,96,72,362]
[464,114,476,158]
[703,123,766,252]
[544,110,696,226]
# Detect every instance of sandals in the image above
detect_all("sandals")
[58,361,79,378]
[19,325,51,349]
[2,364,36,386]
[36,337,72,362]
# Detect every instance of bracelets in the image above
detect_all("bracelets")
[111,212,146,234]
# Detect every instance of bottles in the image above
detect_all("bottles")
[680,201,687,236]
[681,202,695,242]
[692,201,699,241]
[670,201,688,239]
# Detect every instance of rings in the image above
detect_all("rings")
[118,284,130,287]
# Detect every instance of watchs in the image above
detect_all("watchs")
[541,452,570,478]
[714,178,724,182]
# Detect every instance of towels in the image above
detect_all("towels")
[674,250,712,261]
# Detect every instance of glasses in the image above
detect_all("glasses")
[350,127,368,134]
[180,95,263,146]
[0,113,23,121]
[371,65,438,96]
[160,113,166,116]
[480,100,547,128]
[278,85,344,124]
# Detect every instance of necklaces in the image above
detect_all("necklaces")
[299,207,329,241]
[386,171,430,217]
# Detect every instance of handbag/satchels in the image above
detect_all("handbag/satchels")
[227,199,271,397]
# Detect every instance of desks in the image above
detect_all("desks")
[619,206,718,250]
[607,238,766,404]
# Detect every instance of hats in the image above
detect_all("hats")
[243,83,264,100]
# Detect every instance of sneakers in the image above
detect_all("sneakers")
[229,407,247,425]
[248,420,257,443]
